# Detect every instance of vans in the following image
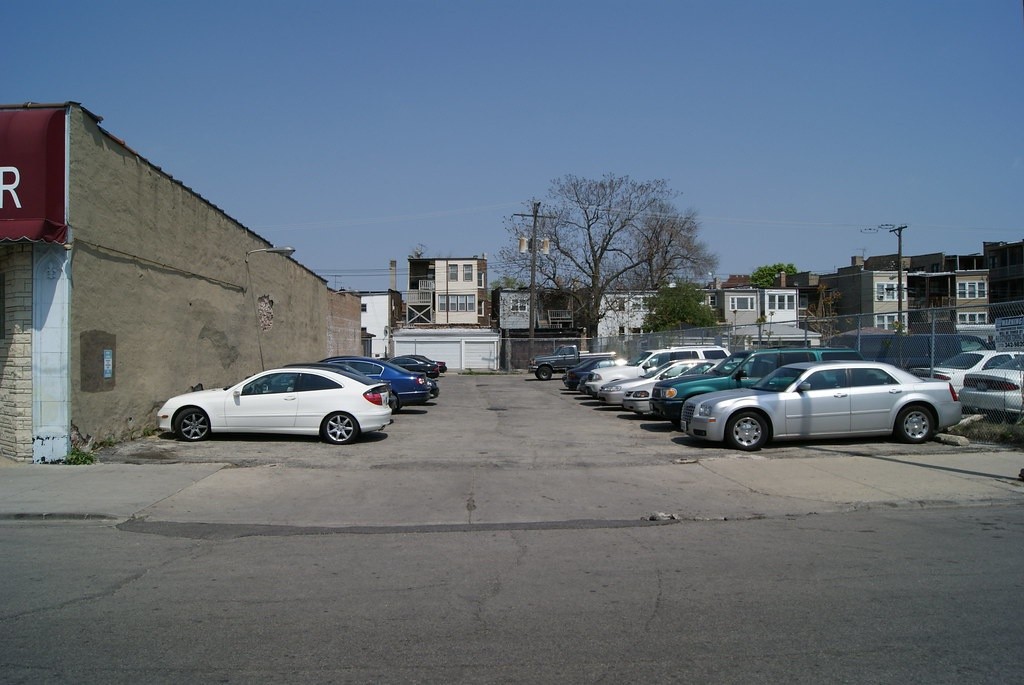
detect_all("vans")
[828,333,994,370]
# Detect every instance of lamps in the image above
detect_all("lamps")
[245,246,296,262]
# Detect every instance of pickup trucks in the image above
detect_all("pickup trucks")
[528,343,616,380]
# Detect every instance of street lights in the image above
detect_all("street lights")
[888,275,903,333]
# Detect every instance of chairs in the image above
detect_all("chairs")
[287,376,295,391]
[837,371,846,388]
[813,374,828,389]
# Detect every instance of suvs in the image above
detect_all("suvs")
[650,349,865,423]
[585,346,733,397]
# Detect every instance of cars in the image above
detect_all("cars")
[958,354,1024,418]
[600,358,749,416]
[155,363,394,445]
[562,357,630,392]
[316,356,439,413]
[681,361,964,451]
[379,355,446,378]
[909,349,1024,397]
